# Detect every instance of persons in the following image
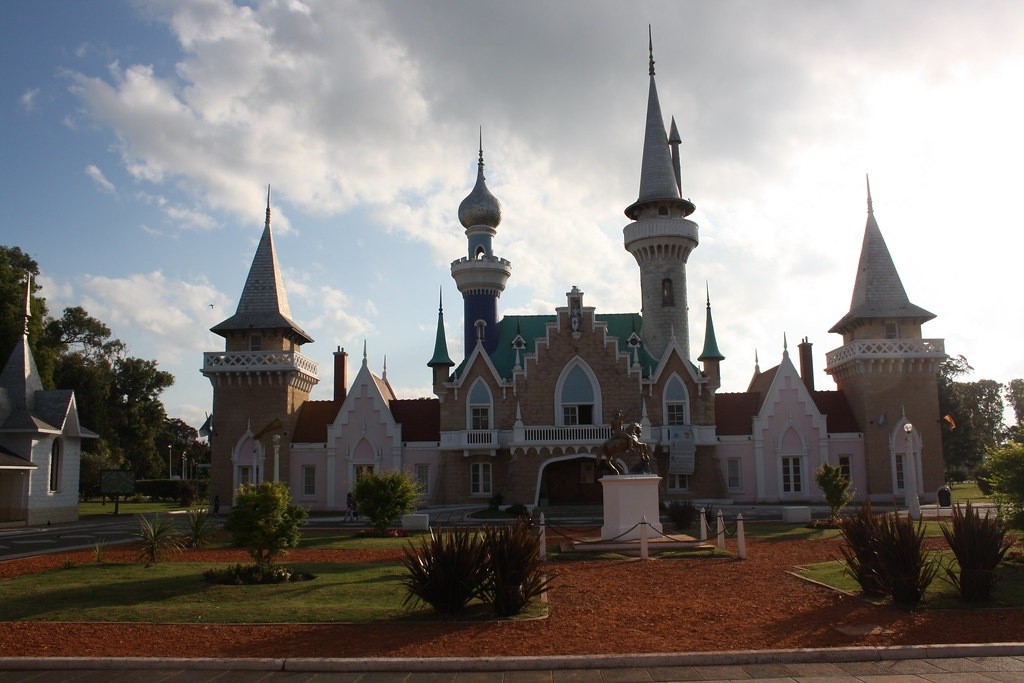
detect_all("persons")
[344,493,359,522]
[213,495,219,518]
[612,408,636,451]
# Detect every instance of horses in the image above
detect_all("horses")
[594,422,653,475]
